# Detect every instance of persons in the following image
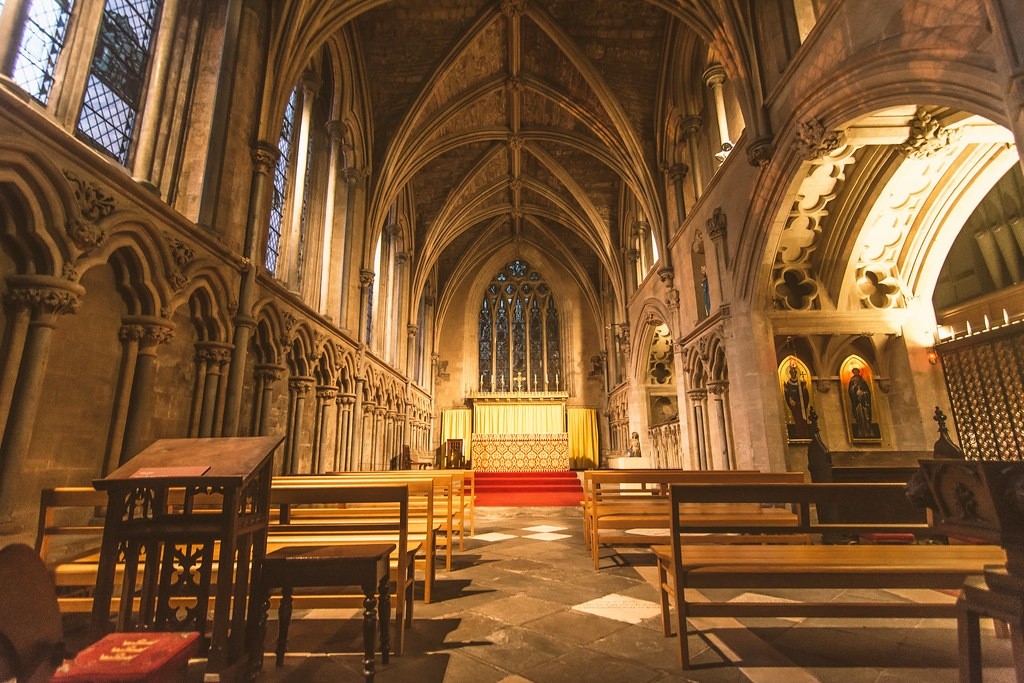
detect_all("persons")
[648,423,680,469]
[629,433,639,454]
[849,368,875,438]
[785,367,809,438]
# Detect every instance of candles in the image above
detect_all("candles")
[480,374,483,382]
[502,375,504,382]
[546,374,548,381]
[534,374,537,382]
[491,374,494,383]
[556,374,558,382]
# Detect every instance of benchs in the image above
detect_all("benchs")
[579,467,1008,673]
[34,468,476,657]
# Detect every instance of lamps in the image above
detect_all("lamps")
[714,142,734,162]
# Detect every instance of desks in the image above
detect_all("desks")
[243,543,396,683]
